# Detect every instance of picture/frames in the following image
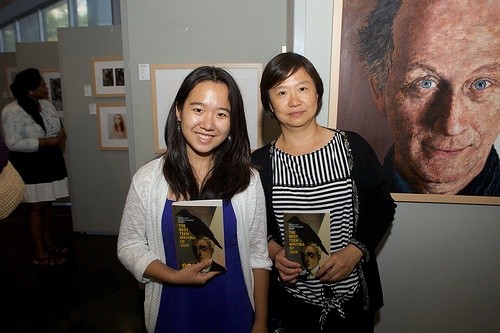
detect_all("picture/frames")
[95,102,129,150]
[38,67,64,121]
[150,63,263,156]
[5,66,17,99]
[91,57,126,98]
[327,0,499,206]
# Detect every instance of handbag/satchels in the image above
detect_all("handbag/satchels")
[0,160,25,221]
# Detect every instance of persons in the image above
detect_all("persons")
[116,67,272,333]
[0,67,69,268]
[249,51,398,332]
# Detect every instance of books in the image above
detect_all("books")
[172,198,226,271]
[283,209,331,280]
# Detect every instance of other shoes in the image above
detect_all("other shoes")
[43,244,69,257]
[32,256,67,267]
